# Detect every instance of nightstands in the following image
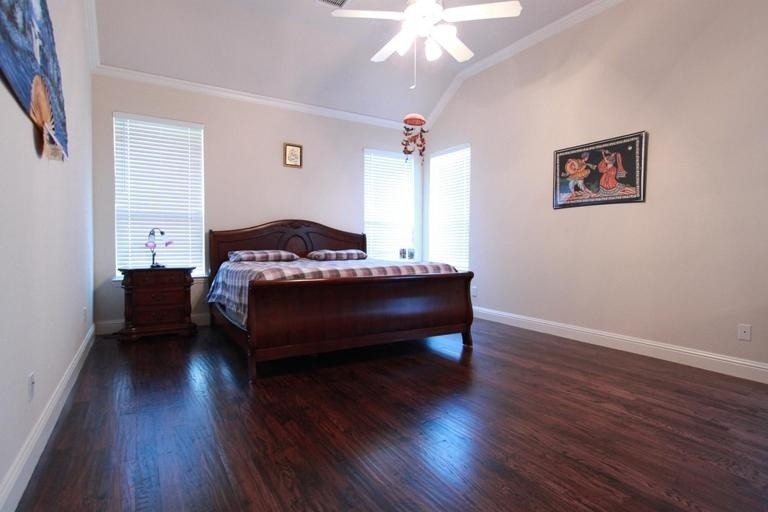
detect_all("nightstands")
[114,263,199,344]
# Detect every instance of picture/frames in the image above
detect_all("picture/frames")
[550,127,649,210]
[281,141,305,169]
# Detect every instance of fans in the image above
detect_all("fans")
[329,2,524,68]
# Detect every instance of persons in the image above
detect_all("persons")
[561,150,597,197]
[598,149,629,195]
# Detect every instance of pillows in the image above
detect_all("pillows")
[305,247,369,262]
[228,249,301,263]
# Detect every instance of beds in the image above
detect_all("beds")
[208,217,477,385]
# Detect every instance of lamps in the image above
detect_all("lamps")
[144,227,175,269]
[396,14,460,65]
